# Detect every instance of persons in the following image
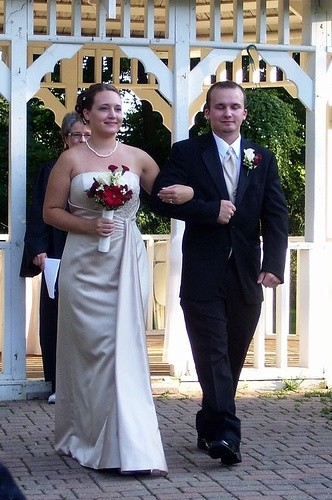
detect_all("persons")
[149,81,289,466]
[43,83,194,474]
[19,111,91,403]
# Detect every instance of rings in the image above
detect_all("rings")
[170,199,173,203]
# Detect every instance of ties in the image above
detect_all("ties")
[223,147,237,204]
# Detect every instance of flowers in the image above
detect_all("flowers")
[83,164,134,252]
[243,147,263,177]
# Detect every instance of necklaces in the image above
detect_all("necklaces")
[86,137,118,158]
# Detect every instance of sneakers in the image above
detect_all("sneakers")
[198,432,208,449]
[208,439,241,464]
[48,392,55,402]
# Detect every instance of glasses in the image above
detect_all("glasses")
[67,132,91,141]
